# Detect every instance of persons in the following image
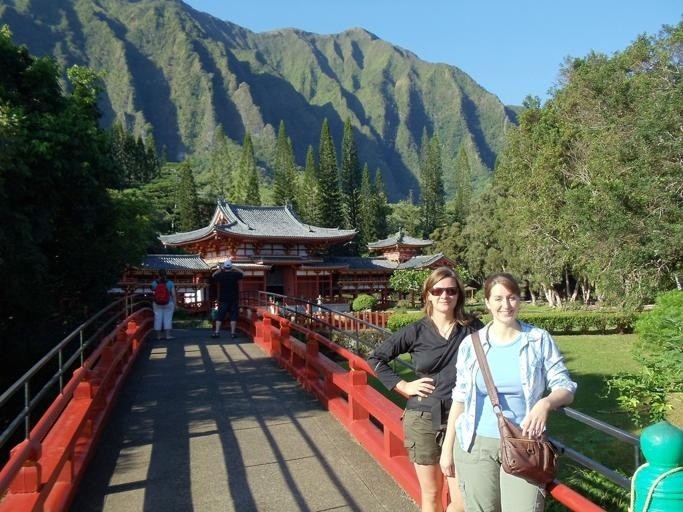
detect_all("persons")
[212,260,244,339]
[151,269,178,336]
[440,272,579,512]
[367,267,485,512]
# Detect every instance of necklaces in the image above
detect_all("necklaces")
[433,316,455,339]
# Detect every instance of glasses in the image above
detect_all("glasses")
[428,287,459,296]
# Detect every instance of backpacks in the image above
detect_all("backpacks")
[154,279,170,305]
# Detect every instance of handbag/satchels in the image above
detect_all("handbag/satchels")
[498,413,559,482]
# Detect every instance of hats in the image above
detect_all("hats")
[223,260,232,268]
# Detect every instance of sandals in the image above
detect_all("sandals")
[212,333,220,337]
